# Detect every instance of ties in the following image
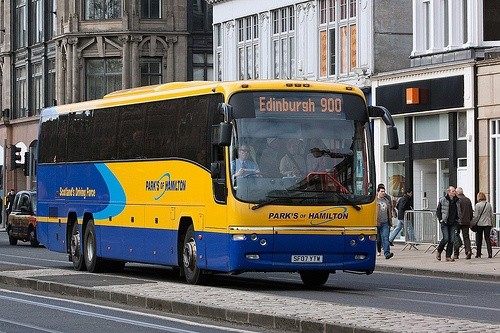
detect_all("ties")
[242,162,246,169]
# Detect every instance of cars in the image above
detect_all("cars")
[6,190,40,247]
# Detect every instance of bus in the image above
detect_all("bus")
[36,78,399,289]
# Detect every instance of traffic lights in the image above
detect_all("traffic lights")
[10,144,21,171]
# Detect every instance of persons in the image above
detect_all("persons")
[232,136,330,177]
[389,188,419,246]
[6,190,17,226]
[21,196,31,212]
[48,128,207,167]
[454,186,473,259]
[377,184,394,260]
[470,191,493,258]
[435,186,458,261]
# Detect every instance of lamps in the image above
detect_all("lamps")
[406,88,419,104]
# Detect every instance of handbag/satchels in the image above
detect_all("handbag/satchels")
[470,224,478,232]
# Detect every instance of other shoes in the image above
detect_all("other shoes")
[455,255,459,259]
[385,253,393,259]
[489,253,492,258]
[389,241,394,247]
[436,251,441,261]
[446,257,455,262]
[377,252,381,256]
[465,251,471,260]
[475,254,481,258]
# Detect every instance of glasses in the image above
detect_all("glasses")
[239,149,250,153]
